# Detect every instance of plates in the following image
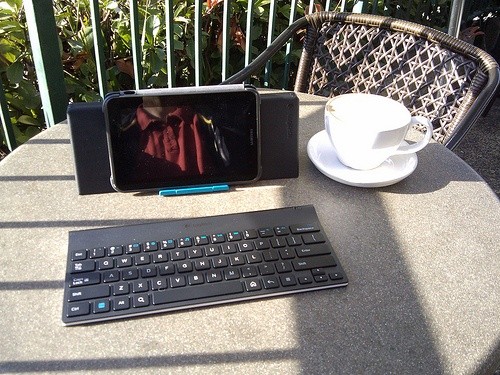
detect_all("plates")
[307,129,419,188]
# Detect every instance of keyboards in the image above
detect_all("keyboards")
[62,203,350,327]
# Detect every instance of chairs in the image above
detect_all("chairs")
[217,12,500,151]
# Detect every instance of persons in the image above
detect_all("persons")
[113,95,219,194]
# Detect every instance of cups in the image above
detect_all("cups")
[324,93,433,171]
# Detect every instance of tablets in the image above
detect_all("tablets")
[104,88,260,193]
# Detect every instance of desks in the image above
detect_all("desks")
[0,88,500,375]
